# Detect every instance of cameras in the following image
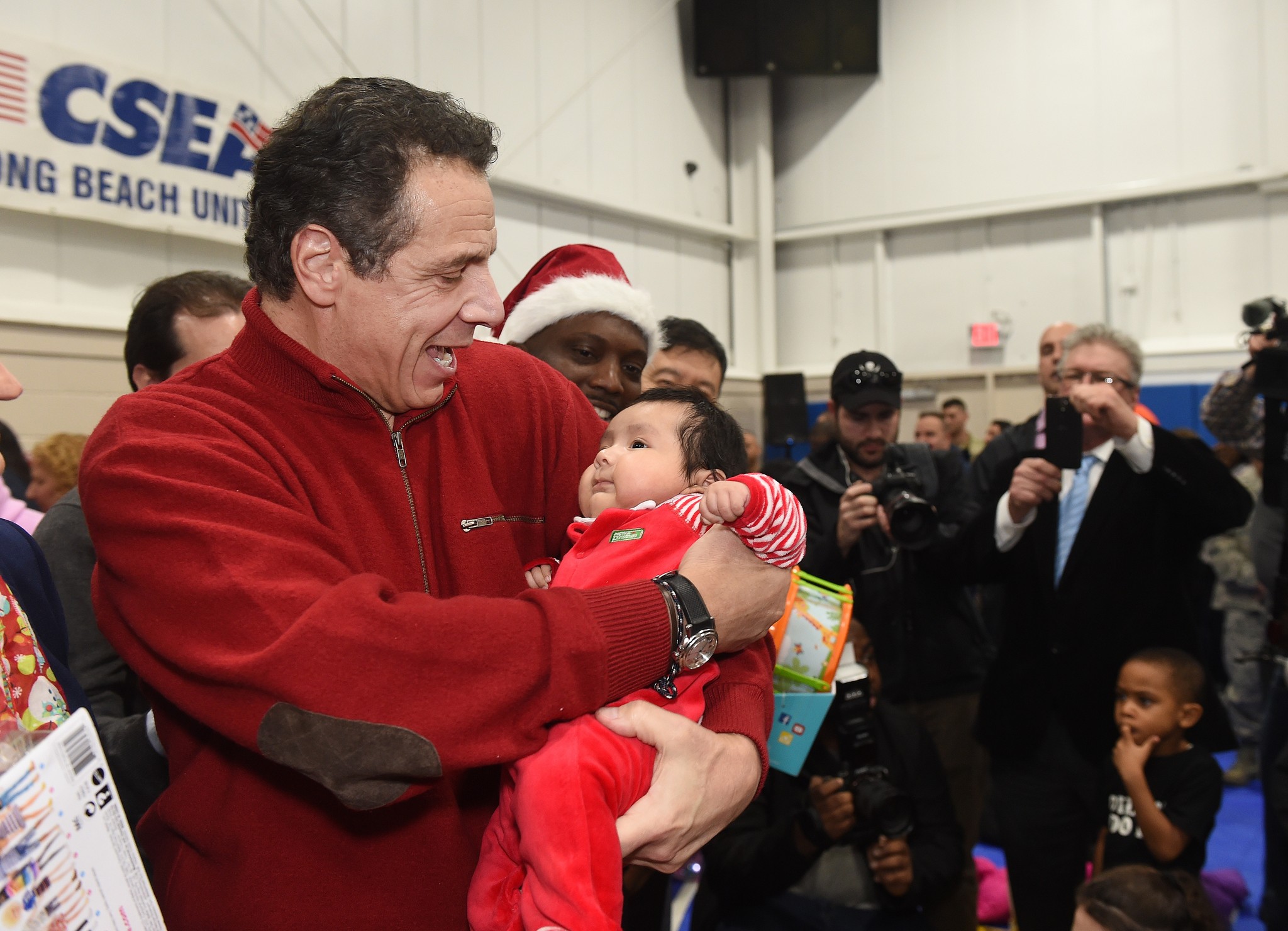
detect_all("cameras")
[826,662,916,843]
[863,469,938,546]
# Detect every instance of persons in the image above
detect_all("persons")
[491,244,654,422]
[464,387,808,931]
[640,294,1288,931]
[1073,865,1234,931]
[80,76,791,931]
[0,364,75,782]
[33,270,254,836]
[1092,646,1222,877]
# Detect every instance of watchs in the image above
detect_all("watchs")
[650,569,718,671]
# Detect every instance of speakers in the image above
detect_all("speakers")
[692,0,881,77]
[761,373,810,445]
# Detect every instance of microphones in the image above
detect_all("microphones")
[1242,300,1271,327]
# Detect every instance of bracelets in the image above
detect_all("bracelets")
[650,578,684,699]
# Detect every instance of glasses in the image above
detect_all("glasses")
[1047,369,1138,394]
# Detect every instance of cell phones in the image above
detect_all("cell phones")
[1045,397,1082,469]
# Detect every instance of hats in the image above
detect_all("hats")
[490,245,669,362]
[830,350,902,412]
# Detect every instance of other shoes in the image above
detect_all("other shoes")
[1223,747,1262,786]
